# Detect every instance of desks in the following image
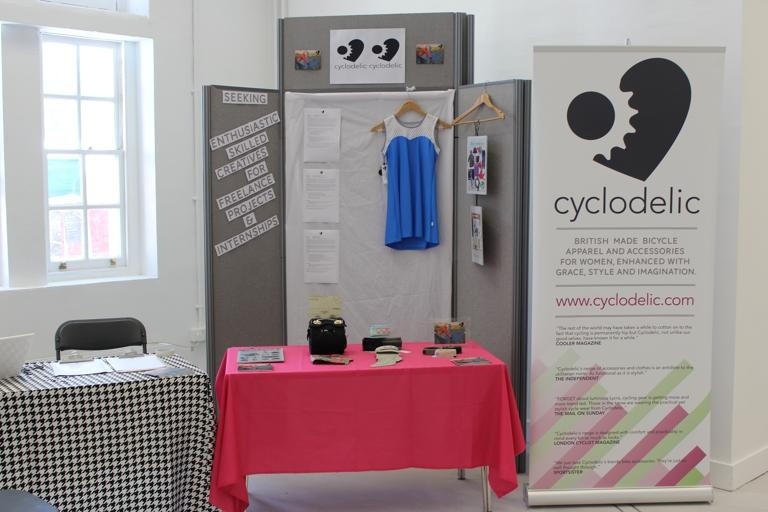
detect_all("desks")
[0,353,212,512]
[212,337,526,512]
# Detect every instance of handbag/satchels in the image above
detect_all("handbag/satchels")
[308,317,347,355]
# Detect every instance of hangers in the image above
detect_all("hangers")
[371,87,452,132]
[453,83,506,126]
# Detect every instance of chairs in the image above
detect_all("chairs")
[55,317,147,360]
